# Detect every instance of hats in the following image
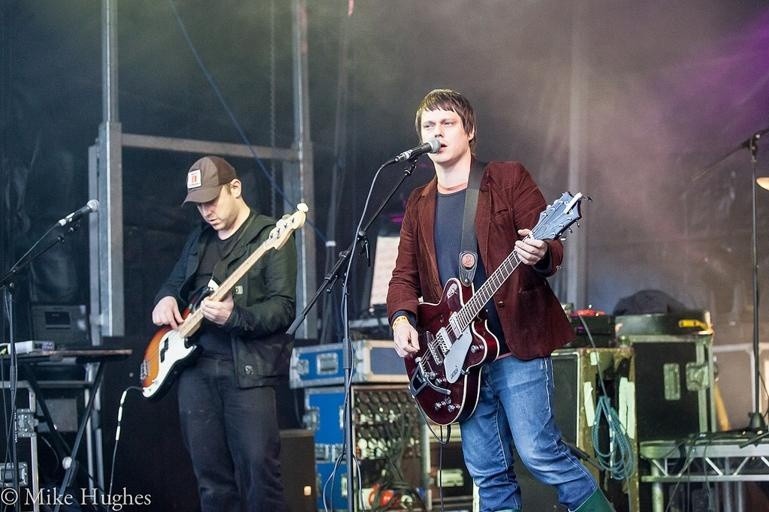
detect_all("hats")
[180,156,236,209]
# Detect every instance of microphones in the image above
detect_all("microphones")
[395,138,441,161]
[57,198,99,226]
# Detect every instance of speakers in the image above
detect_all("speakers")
[279,428,317,512]
[509,346,640,512]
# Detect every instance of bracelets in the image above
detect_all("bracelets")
[392,315,409,330]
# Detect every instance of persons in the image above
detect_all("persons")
[150,155,297,512]
[385,88,614,512]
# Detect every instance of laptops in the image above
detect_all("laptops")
[27,302,121,349]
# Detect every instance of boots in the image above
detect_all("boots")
[567,487,618,512]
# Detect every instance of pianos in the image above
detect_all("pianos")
[350,305,615,348]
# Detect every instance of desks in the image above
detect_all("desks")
[0,347,133,510]
[640,433,768,512]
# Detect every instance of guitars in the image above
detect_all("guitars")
[758,178,769,187]
[405,190,581,426]
[139,203,309,403]
